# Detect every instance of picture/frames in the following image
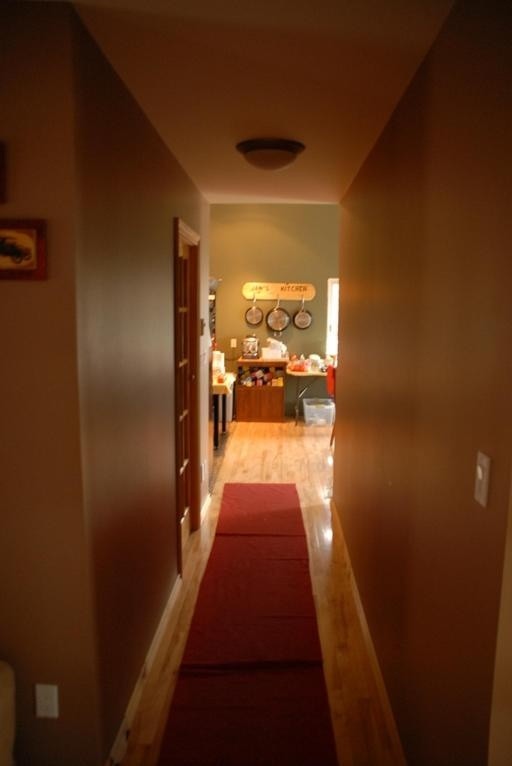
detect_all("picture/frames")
[0,216,49,281]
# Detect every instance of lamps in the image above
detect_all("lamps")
[235,138,305,171]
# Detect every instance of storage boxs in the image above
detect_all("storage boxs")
[301,398,335,425]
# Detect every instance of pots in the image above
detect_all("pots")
[293,295,313,330]
[245,294,263,326]
[266,297,292,338]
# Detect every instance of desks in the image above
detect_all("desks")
[284,362,336,427]
[212,371,237,451]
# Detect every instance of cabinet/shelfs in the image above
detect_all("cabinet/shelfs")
[233,356,287,423]
